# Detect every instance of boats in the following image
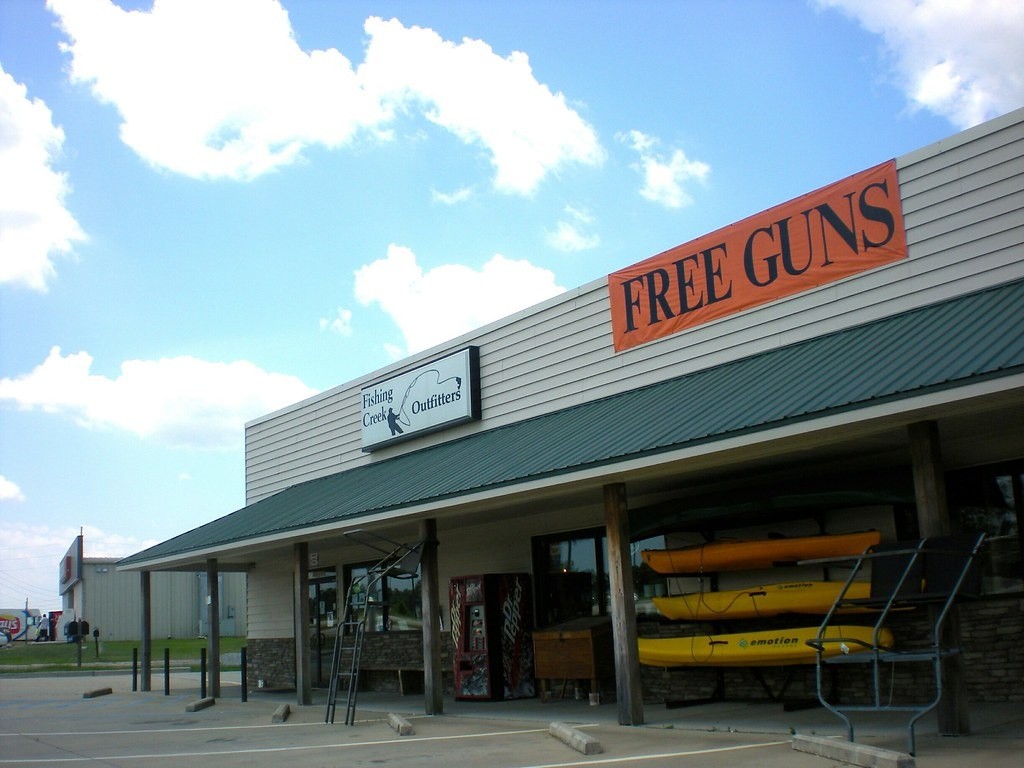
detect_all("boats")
[652,579,923,623]
[640,528,882,574]
[636,625,900,669]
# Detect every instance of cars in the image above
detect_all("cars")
[0,626,12,647]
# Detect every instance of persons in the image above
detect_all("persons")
[367,597,378,632]
[35,614,49,642]
